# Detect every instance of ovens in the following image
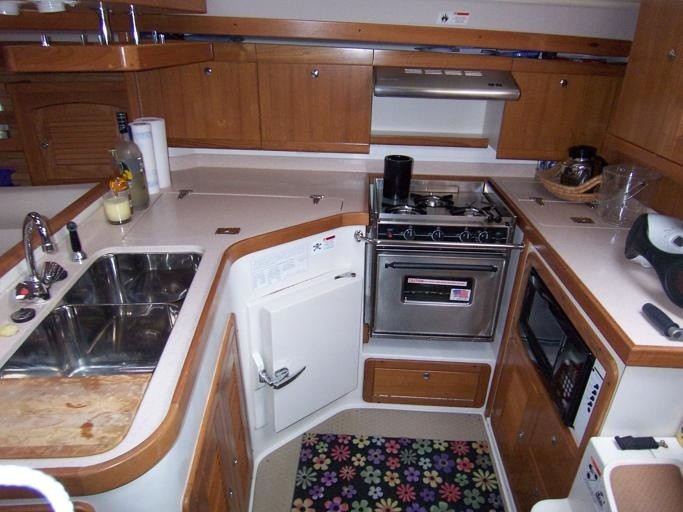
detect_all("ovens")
[372,246,508,345]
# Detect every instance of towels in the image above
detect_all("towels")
[0,167,20,188]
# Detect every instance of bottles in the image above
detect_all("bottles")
[560,144,609,194]
[66,220,87,261]
[114,112,150,210]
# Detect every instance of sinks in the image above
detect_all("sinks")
[0,227,23,256]
[57,243,211,304]
[1,181,101,228]
[0,300,180,378]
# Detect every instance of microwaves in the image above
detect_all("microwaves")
[512,271,605,436]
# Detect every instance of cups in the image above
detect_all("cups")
[101,188,131,224]
[383,155,413,201]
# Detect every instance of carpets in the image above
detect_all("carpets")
[286,425,507,512]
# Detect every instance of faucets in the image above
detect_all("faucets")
[22,212,53,282]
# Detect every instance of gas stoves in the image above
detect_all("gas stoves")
[371,178,515,249]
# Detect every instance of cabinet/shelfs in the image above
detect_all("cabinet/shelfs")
[365,364,481,408]
[497,63,622,166]
[133,54,260,153]
[14,77,134,188]
[182,335,256,511]
[479,338,579,512]
[607,2,682,167]
[259,57,370,158]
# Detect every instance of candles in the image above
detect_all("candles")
[99,189,132,225]
